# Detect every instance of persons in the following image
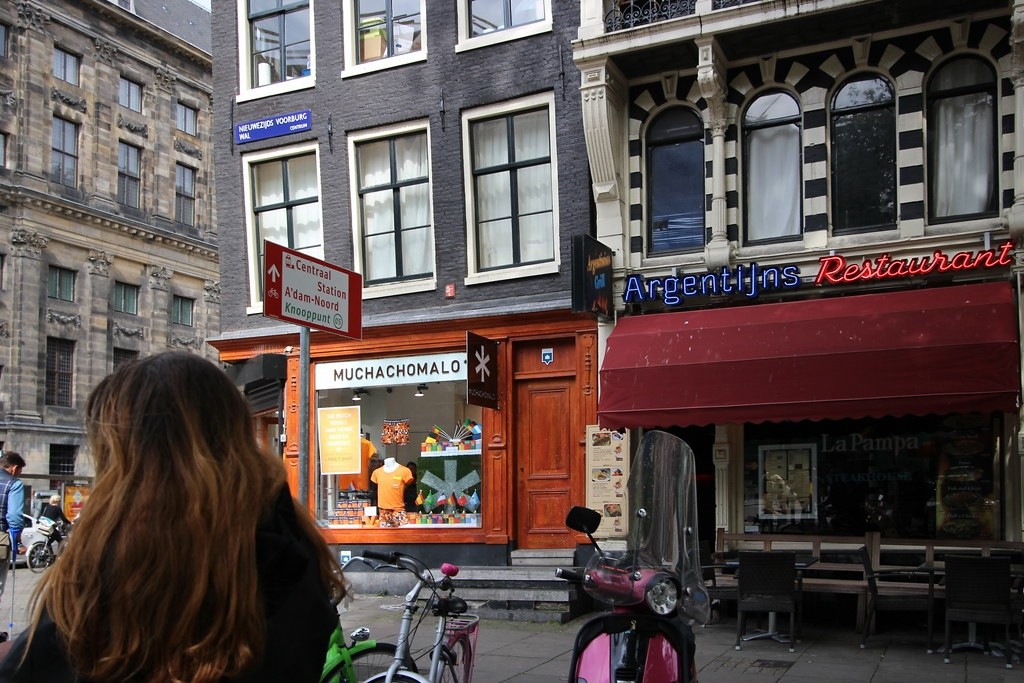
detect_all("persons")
[1,348,342,683]
[339,426,379,491]
[403,461,418,511]
[1,451,27,643]
[37,494,74,556]
[369,457,413,510]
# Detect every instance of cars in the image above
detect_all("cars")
[5,512,68,565]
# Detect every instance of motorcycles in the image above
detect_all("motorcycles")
[553,427,719,683]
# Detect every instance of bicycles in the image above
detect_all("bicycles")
[314,556,418,683]
[359,549,478,683]
[24,521,69,574]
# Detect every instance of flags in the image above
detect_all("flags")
[436,492,449,506]
[469,491,481,506]
[457,493,468,507]
[424,490,437,506]
[426,417,482,442]
[448,491,460,506]
[414,491,425,506]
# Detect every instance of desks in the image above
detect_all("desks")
[726,554,820,642]
[920,557,1024,663]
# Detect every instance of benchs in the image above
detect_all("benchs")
[866,532,1024,631]
[712,526,873,631]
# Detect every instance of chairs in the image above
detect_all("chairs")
[855,545,935,655]
[940,553,1021,670]
[732,551,806,652]
[695,548,741,629]
